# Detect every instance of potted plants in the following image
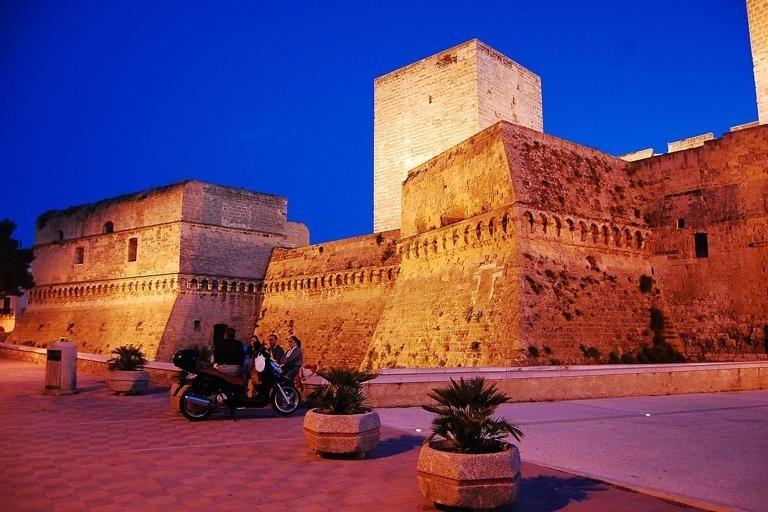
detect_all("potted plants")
[414,375,525,511]
[303,364,382,461]
[169,344,211,412]
[104,344,150,395]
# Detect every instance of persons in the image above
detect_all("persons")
[247,335,261,365]
[277,335,304,387]
[263,333,284,366]
[213,327,246,377]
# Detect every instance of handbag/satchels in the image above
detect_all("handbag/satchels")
[254,354,266,372]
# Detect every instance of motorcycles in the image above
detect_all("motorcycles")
[170,334,301,422]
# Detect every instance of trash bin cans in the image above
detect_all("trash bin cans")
[44,342,79,396]
